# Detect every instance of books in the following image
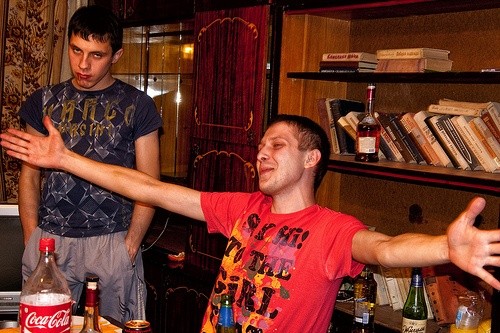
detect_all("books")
[317,48,500,326]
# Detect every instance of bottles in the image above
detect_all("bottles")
[216,295,235,333]
[353,265,377,333]
[20,238,72,333]
[79,276,103,333]
[355,83,381,163]
[402,267,428,333]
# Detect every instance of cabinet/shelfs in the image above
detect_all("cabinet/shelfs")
[261,0,500,333]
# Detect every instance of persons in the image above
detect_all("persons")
[0,114,500,333]
[19,6,164,323]
